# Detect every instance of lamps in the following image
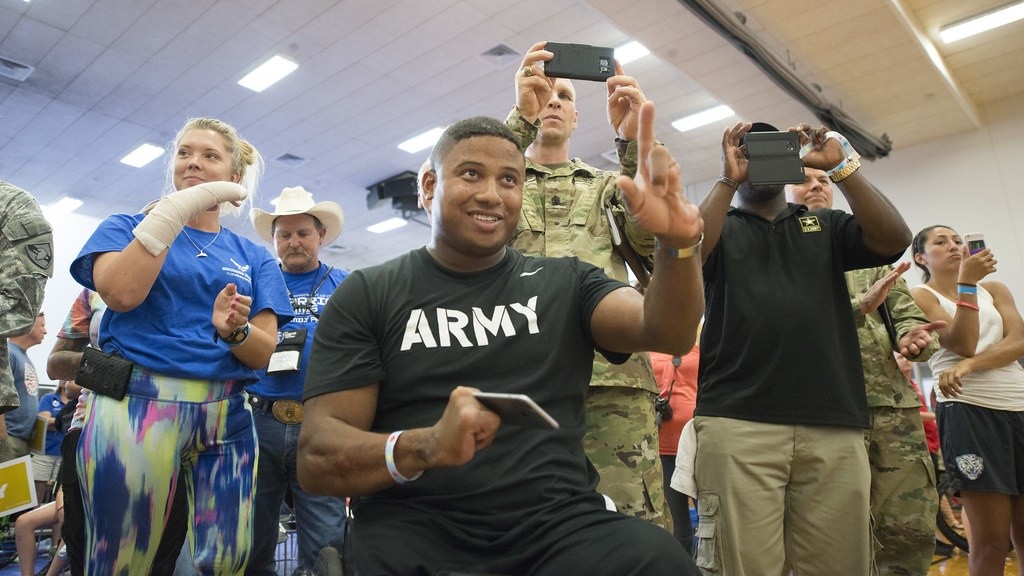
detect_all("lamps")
[365,170,424,221]
[940,0,1024,44]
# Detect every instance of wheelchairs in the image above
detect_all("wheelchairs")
[293,492,349,576]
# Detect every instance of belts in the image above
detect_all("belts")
[247,393,304,425]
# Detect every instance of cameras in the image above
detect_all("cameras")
[655,396,675,428]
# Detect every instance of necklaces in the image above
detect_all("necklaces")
[182,224,221,258]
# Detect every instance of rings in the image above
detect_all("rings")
[523,66,533,77]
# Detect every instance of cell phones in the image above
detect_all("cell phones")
[964,232,988,257]
[474,390,561,432]
[742,130,807,190]
[543,40,616,84]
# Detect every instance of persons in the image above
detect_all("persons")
[16,399,69,576]
[45,285,107,575]
[417,157,431,227]
[1,181,52,465]
[8,310,46,459]
[250,186,353,575]
[69,119,294,576]
[57,379,90,576]
[40,377,73,457]
[296,42,1024,576]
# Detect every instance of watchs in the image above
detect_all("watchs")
[213,321,249,346]
[829,159,861,183]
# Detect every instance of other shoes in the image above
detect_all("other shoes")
[244,565,280,576]
[954,524,965,529]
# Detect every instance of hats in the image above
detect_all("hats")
[738,122,779,147]
[249,187,345,249]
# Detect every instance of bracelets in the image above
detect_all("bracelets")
[655,233,704,259]
[385,430,423,484]
[717,174,737,189]
[957,283,977,294]
[957,301,979,311]
